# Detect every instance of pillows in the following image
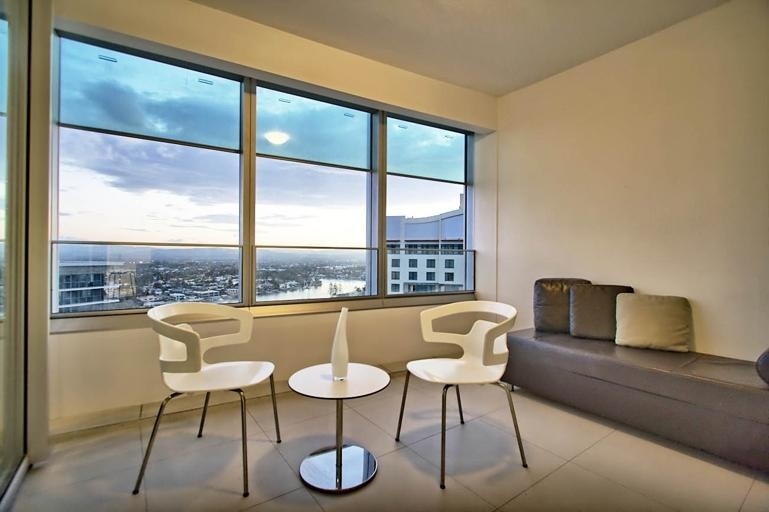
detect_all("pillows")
[756,347,769,385]
[531,277,691,352]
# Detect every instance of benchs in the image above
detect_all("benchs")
[502,328,769,477]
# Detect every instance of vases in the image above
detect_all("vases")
[331,306,349,381]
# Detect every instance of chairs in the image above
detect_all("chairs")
[132,301,282,496]
[394,300,529,489]
